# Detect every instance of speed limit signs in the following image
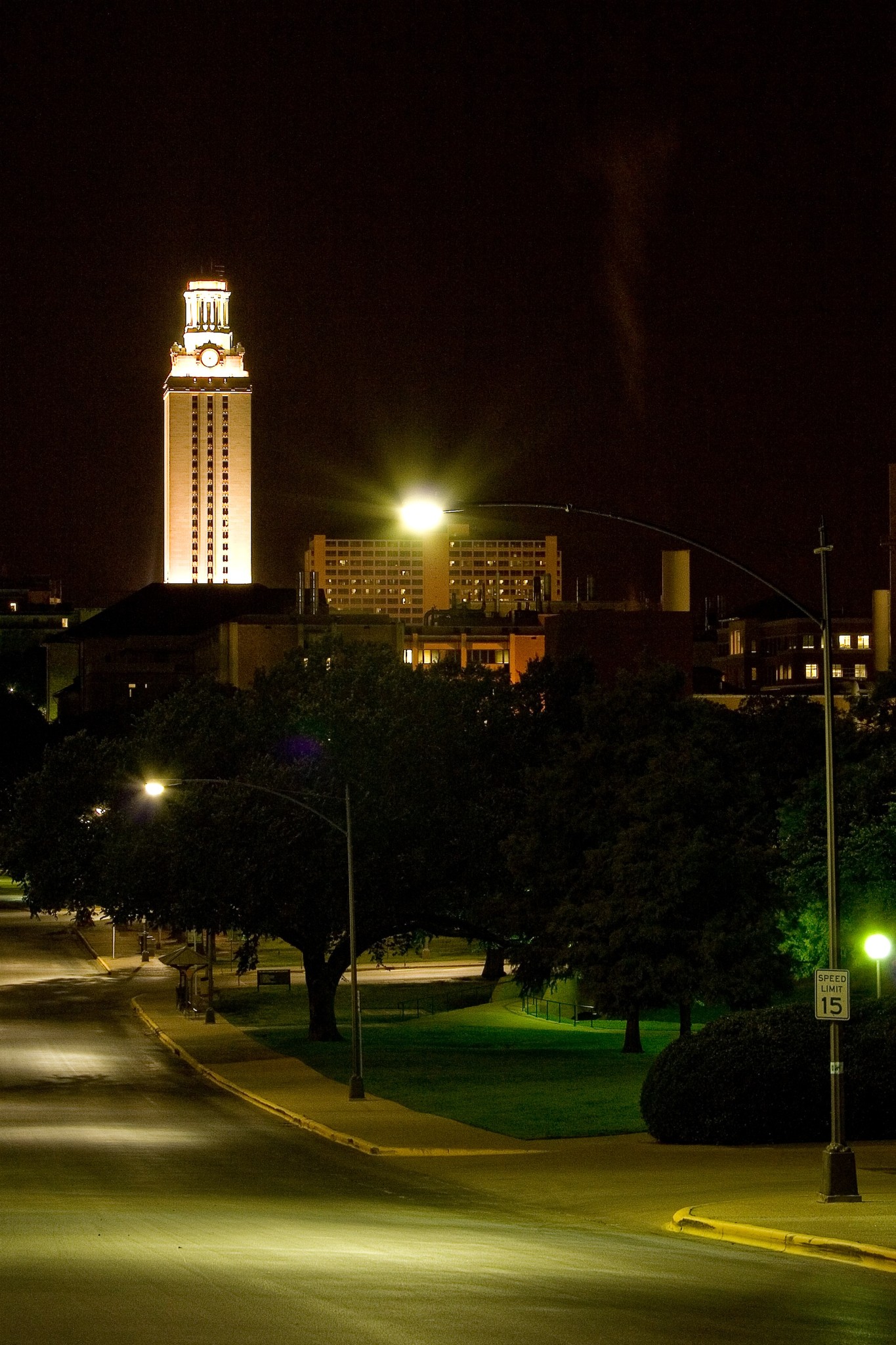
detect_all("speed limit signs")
[815,968,850,1023]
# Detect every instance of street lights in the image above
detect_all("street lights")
[141,778,367,1100]
[865,933,890,999]
[399,496,866,1206]
